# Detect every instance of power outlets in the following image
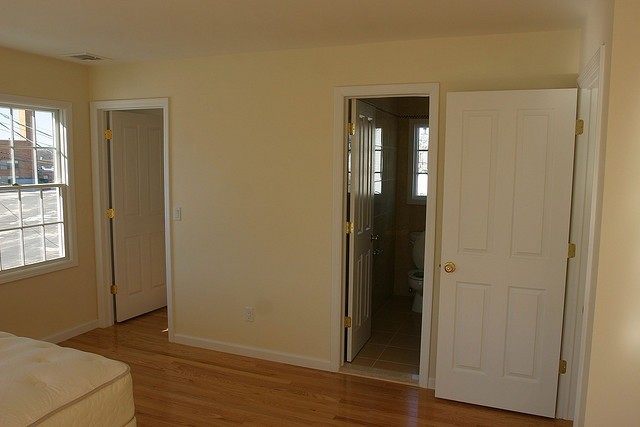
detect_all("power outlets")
[244,307,254,322]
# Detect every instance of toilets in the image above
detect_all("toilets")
[407,230,425,313]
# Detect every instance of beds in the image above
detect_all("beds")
[0,332,137,427]
